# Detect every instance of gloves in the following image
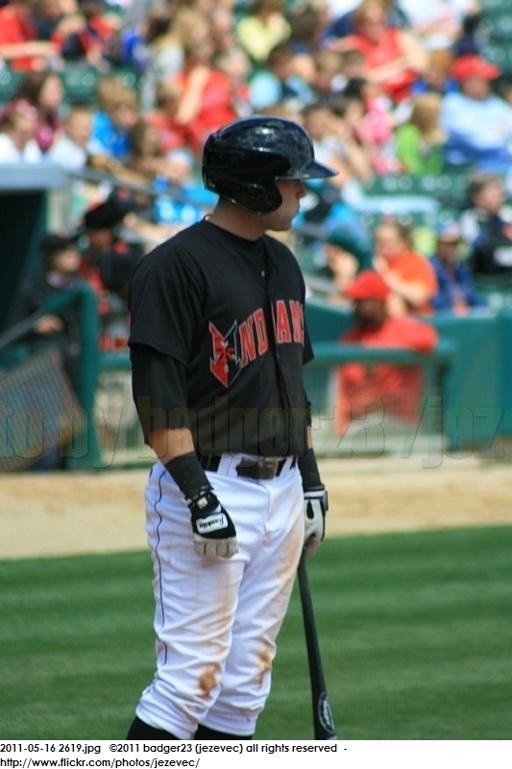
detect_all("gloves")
[188,491,237,557]
[304,486,328,559]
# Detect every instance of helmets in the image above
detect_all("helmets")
[204,119,339,216]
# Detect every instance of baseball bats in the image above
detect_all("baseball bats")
[296,547,338,740]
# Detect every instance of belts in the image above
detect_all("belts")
[197,452,295,479]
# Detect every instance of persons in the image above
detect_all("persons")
[126,117,335,740]
